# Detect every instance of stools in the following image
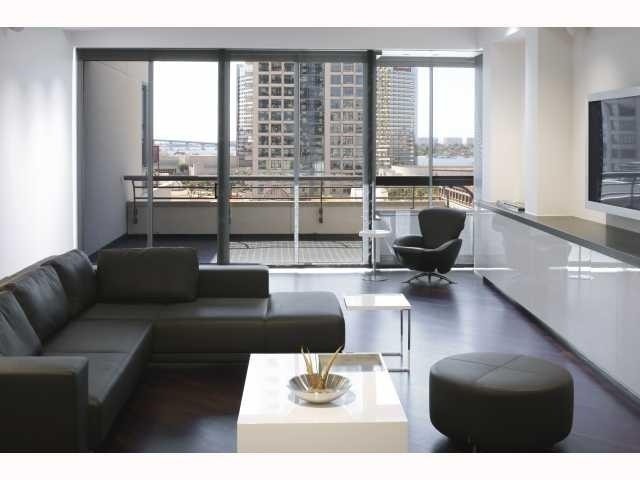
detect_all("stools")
[426,351,576,454]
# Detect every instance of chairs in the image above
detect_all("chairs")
[388,205,469,288]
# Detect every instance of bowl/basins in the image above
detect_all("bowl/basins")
[285,372,351,404]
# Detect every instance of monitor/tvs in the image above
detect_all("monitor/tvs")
[584,86,639,220]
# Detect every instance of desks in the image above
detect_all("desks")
[357,227,393,283]
[339,291,413,376]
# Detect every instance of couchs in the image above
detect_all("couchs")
[0,257,157,452]
[0,242,347,372]
